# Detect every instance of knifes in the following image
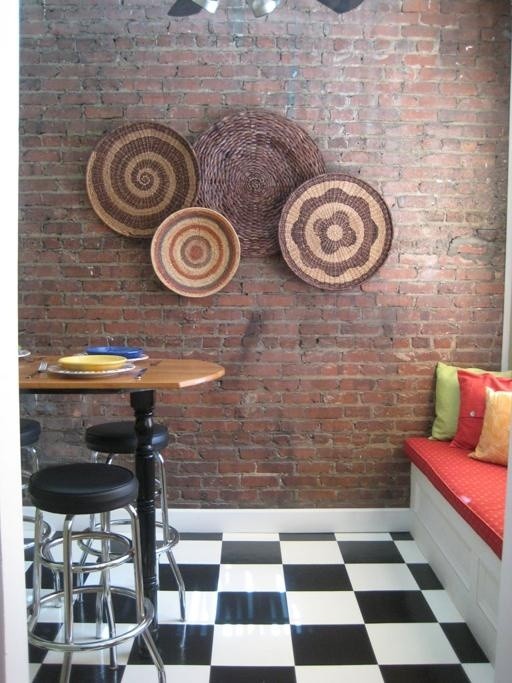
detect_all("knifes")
[137,359,162,380]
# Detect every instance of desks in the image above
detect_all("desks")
[19,355,227,658]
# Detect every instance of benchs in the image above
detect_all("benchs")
[402,438,507,671]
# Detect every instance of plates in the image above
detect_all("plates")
[18,348,30,358]
[47,347,150,376]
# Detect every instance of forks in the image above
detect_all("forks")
[25,361,46,380]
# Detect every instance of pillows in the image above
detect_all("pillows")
[429,361,512,470]
